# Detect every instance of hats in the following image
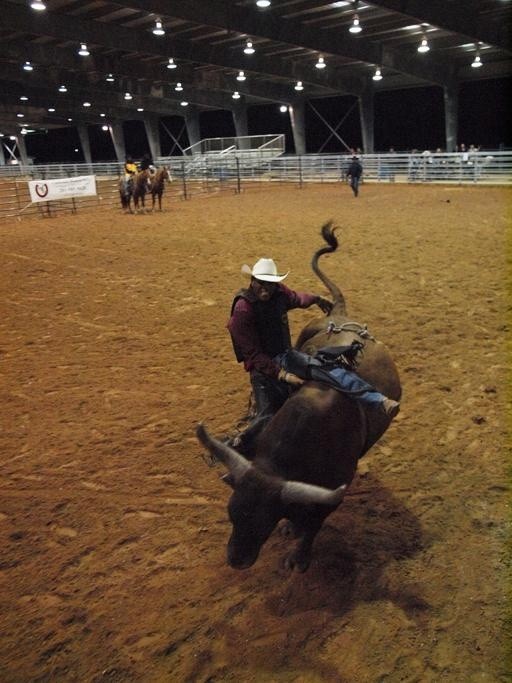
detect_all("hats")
[351,155,359,160]
[239,255,292,284]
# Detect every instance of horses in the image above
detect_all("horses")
[117,167,152,215]
[136,165,173,211]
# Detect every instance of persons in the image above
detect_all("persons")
[346,156,363,198]
[140,152,156,188]
[348,144,487,186]
[123,154,138,198]
[208,256,402,447]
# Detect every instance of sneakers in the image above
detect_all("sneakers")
[381,396,400,418]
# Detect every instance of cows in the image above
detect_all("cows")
[193,216,404,577]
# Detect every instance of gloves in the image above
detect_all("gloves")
[276,365,305,389]
[318,294,336,316]
